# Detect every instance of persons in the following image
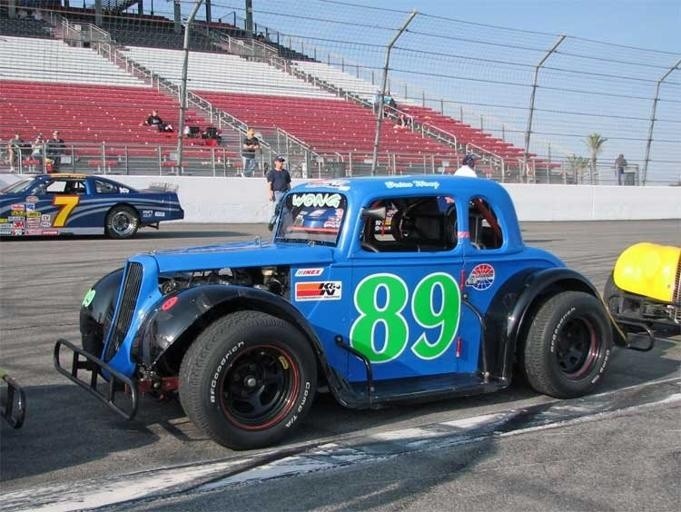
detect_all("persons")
[30,135,46,160]
[241,128,261,177]
[46,130,67,172]
[455,155,478,178]
[385,90,397,112]
[145,108,162,126]
[8,132,27,171]
[266,157,293,231]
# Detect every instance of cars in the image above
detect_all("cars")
[0,170,187,240]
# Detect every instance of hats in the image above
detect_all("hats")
[274,156,285,162]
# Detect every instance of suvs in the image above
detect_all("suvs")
[597,239,680,335]
[47,166,660,444]
